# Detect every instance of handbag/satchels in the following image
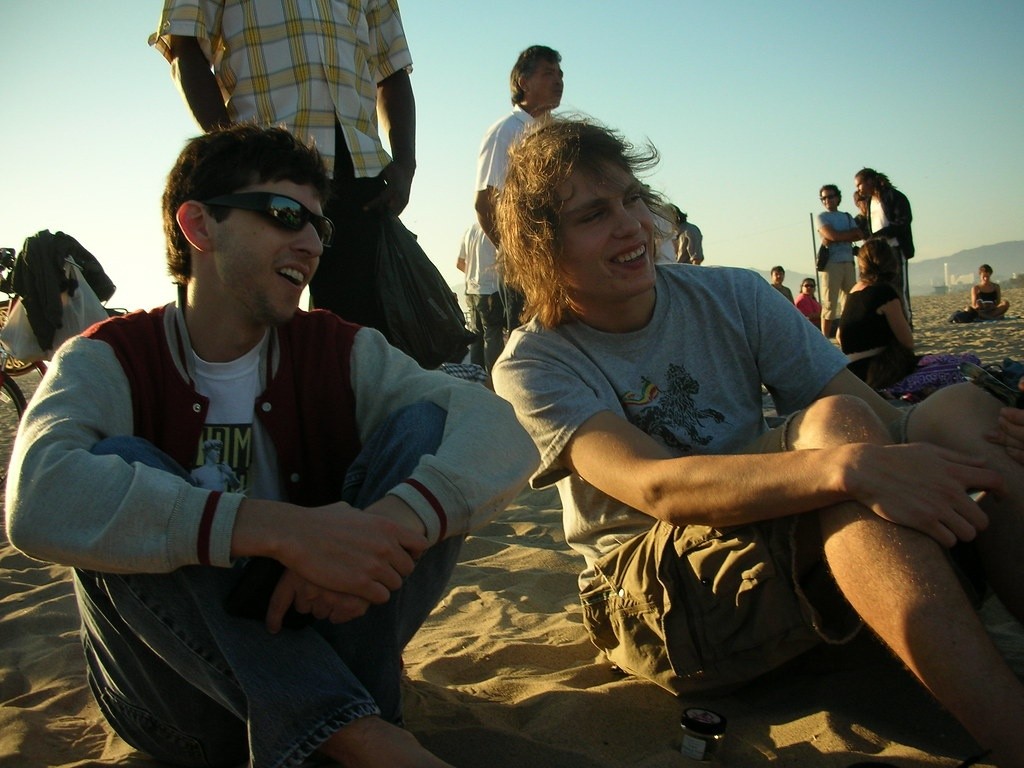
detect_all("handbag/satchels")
[377,211,478,372]
[817,244,830,271]
[0,256,110,362]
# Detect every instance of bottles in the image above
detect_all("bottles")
[674,708,726,761]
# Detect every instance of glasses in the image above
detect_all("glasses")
[196,192,335,249]
[803,284,815,288]
[820,194,837,201]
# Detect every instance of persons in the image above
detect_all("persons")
[490,109,1024,767]
[6,119,542,768]
[458,44,1009,403]
[147,0,476,373]
